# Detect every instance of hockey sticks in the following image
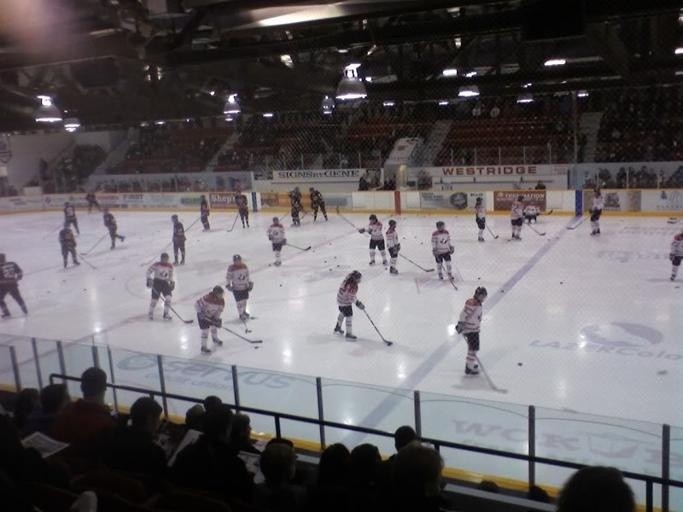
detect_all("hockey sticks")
[460,331,509,393]
[525,221,546,237]
[151,285,194,324]
[227,209,240,232]
[336,205,368,237]
[363,308,393,344]
[205,317,263,344]
[566,214,593,230]
[142,241,173,267]
[485,223,499,240]
[285,244,311,251]
[437,257,459,291]
[79,231,111,256]
[398,253,434,273]
[231,289,252,333]
[75,248,99,270]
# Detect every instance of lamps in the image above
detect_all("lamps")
[33,95,61,122]
[516,92,534,105]
[224,95,242,114]
[457,83,481,98]
[335,63,368,99]
[63,115,82,132]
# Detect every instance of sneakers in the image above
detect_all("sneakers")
[324,215,327,221]
[201,347,211,352]
[239,312,249,321]
[243,223,249,228]
[274,262,281,266]
[512,235,521,239]
[120,236,124,242]
[65,259,80,268]
[214,337,222,344]
[671,274,675,280]
[313,216,316,221]
[290,221,300,226]
[478,237,485,241]
[369,260,375,264]
[1,310,9,316]
[383,260,398,273]
[110,246,113,250]
[590,228,600,235]
[148,313,172,319]
[22,307,27,313]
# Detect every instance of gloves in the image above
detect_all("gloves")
[450,246,454,254]
[227,284,233,292]
[669,254,675,260]
[217,320,223,327]
[169,282,175,291]
[455,321,463,333]
[356,299,364,309]
[146,279,153,287]
[433,249,437,256]
[359,228,364,233]
[199,312,204,320]
[247,283,252,291]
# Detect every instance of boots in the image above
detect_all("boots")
[347,332,357,338]
[465,364,478,374]
[438,273,453,280]
[334,323,344,333]
[173,259,184,265]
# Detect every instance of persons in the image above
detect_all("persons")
[668,229,683,281]
[455,286,489,377]
[0,366,446,512]
[357,86,682,194]
[2,106,357,195]
[334,271,366,343]
[0,252,27,318]
[555,463,631,509]
[58,187,328,268]
[587,186,605,235]
[146,253,253,352]
[354,195,540,285]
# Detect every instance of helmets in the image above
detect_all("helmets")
[273,218,277,224]
[352,272,361,281]
[436,221,444,229]
[475,287,486,299]
[233,255,240,263]
[213,286,222,297]
[161,254,169,264]
[289,187,314,196]
[518,195,523,201]
[370,214,396,227]
[170,215,178,223]
[476,197,482,204]
